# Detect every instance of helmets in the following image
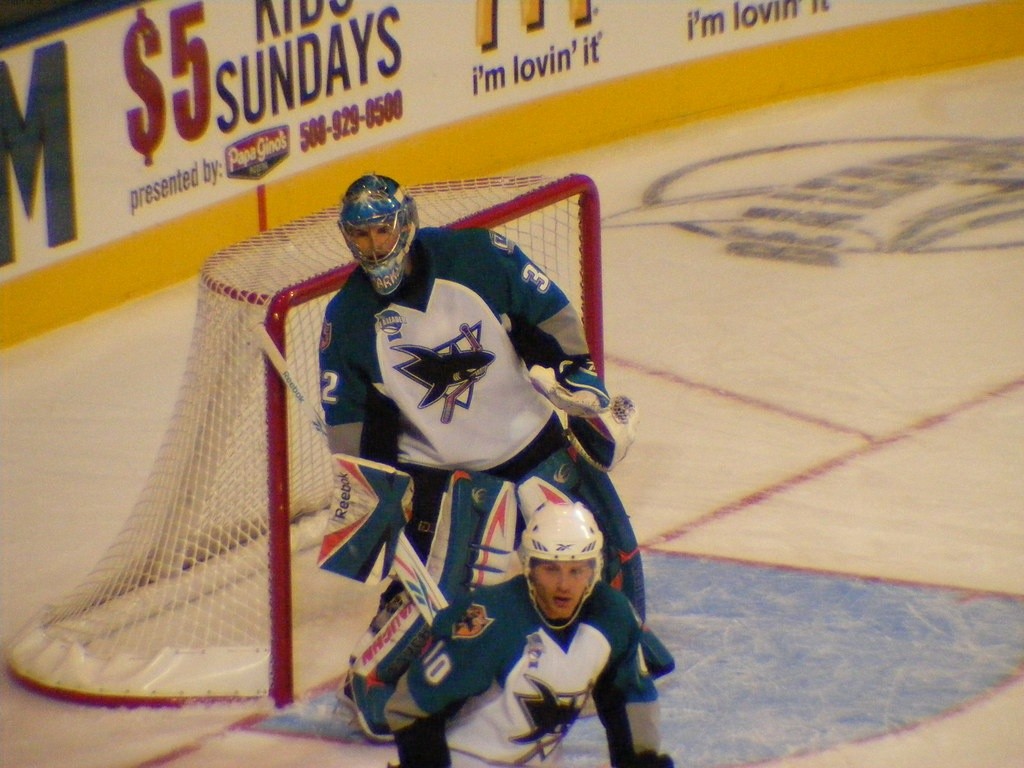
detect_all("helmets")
[337,175,418,296]
[521,501,603,563]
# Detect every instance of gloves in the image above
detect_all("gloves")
[627,750,674,768]
[393,713,451,768]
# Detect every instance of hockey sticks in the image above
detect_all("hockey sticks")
[246,313,453,630]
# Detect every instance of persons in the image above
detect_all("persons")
[384,499,673,768]
[319,174,646,744]
